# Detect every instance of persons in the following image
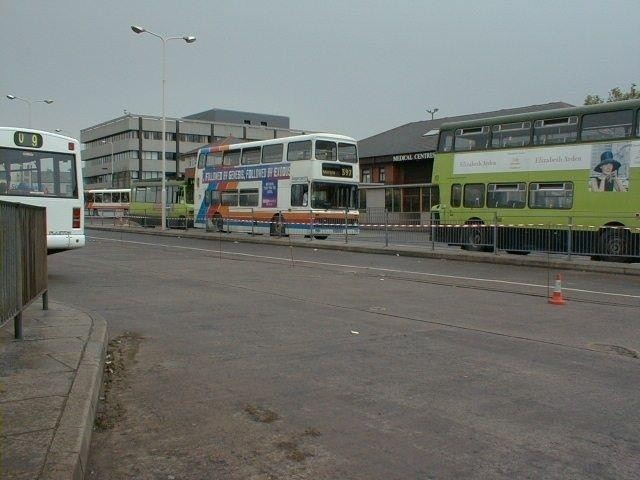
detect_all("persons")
[590,150,627,192]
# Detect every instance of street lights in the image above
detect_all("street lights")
[131,25,197,229]
[6,95,53,125]
[101,139,114,188]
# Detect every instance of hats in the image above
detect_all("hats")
[593,151,621,172]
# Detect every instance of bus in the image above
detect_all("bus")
[87,188,131,219]
[127,180,193,229]
[1,127,85,254]
[193,133,360,240]
[430,102,640,262]
